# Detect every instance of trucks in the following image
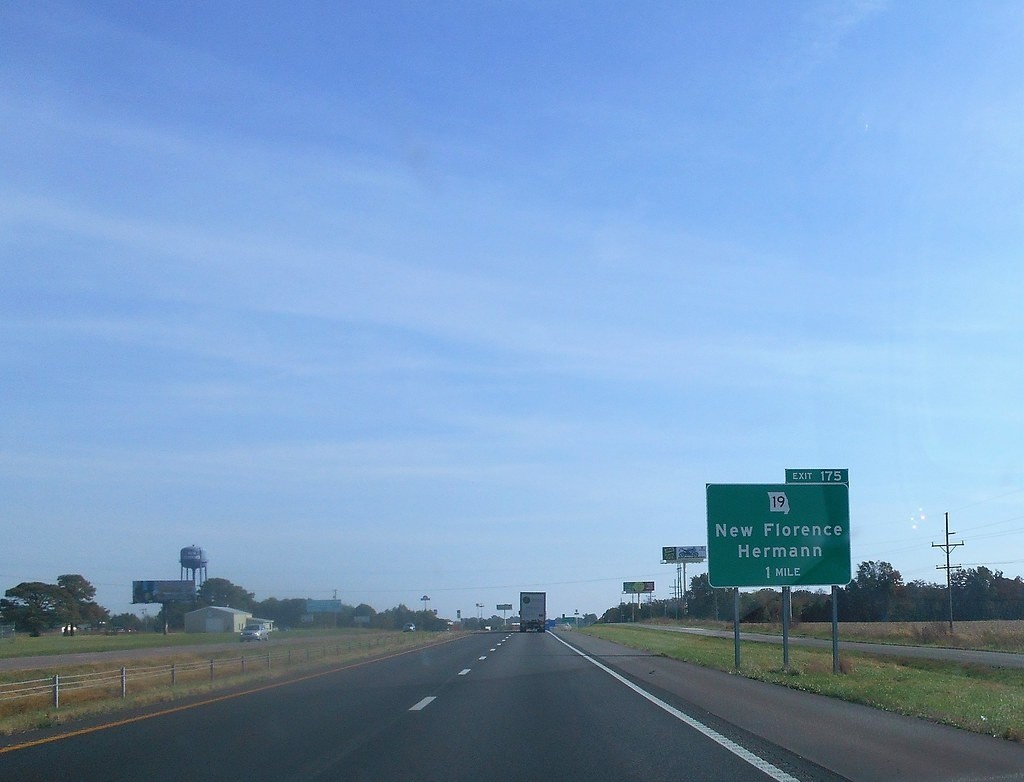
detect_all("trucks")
[519,591,547,633]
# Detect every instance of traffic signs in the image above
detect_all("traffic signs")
[707,482,851,588]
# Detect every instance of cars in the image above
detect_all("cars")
[403,623,416,632]
[240,623,269,642]
